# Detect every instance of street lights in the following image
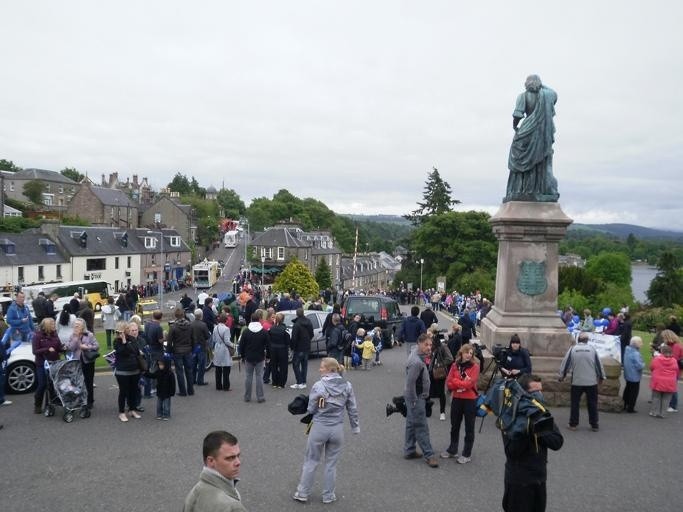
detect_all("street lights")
[420,258,424,292]
[420,259,424,290]
[261,256,265,285]
[261,256,265,284]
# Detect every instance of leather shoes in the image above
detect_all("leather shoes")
[118,413,129,422]
[627,410,638,413]
[129,411,142,419]
[176,391,194,397]
[143,394,155,399]
[217,386,233,391]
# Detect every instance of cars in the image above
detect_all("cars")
[0,339,39,393]
[276,309,332,364]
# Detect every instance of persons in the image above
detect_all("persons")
[167,308,197,397]
[305,283,398,310]
[448,324,461,356]
[440,344,480,464]
[47,294,60,320]
[185,302,195,321]
[7,291,36,342]
[290,308,314,389]
[212,315,236,391]
[419,304,438,329]
[211,294,218,306]
[203,297,218,349]
[184,303,195,323]
[65,318,99,410]
[0,312,13,406]
[142,275,186,297]
[504,74,561,203]
[190,308,210,386]
[70,299,78,314]
[32,318,66,415]
[254,309,263,322]
[348,313,361,337]
[499,334,532,391]
[262,281,304,317]
[399,284,447,312]
[179,293,192,310]
[56,303,77,345]
[143,356,175,421]
[426,327,454,421]
[231,314,244,344]
[668,315,682,336]
[293,357,360,504]
[101,297,121,350]
[447,290,493,320]
[117,283,141,320]
[113,322,141,422]
[622,336,645,414]
[232,270,261,311]
[326,314,346,366]
[652,321,668,351]
[373,327,382,366]
[649,345,679,418]
[500,373,564,512]
[457,309,477,345]
[351,328,365,369]
[404,333,440,468]
[355,335,377,370]
[129,322,154,399]
[262,312,275,385]
[183,430,248,512]
[660,329,682,413]
[238,314,272,404]
[144,310,166,399]
[219,307,232,328]
[268,313,290,390]
[558,333,606,433]
[398,307,425,361]
[31,291,47,324]
[558,299,632,346]
[78,299,95,333]
[198,289,208,307]
[321,304,346,354]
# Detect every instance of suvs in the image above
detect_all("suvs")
[341,295,407,348]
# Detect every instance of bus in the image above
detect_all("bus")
[191,257,218,289]
[0,297,13,321]
[225,231,240,247]
[19,280,115,320]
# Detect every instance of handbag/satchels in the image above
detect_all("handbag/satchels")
[81,332,101,365]
[217,325,236,357]
[455,362,490,417]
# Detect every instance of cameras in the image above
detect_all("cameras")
[491,344,509,364]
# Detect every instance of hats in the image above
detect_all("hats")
[511,333,520,343]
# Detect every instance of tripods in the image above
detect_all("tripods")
[479,359,503,433]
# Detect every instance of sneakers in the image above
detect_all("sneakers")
[439,413,446,421]
[592,424,600,432]
[296,383,307,389]
[197,381,209,385]
[291,491,308,503]
[155,416,169,421]
[289,384,297,389]
[425,457,438,468]
[0,400,13,406]
[322,494,337,504]
[402,450,423,459]
[566,423,578,430]
[244,396,250,402]
[456,456,472,464]
[648,412,665,419]
[273,383,287,389]
[34,404,42,414]
[258,398,266,403]
[440,451,460,459]
[666,407,679,413]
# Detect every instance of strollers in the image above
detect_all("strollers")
[42,343,91,422]
